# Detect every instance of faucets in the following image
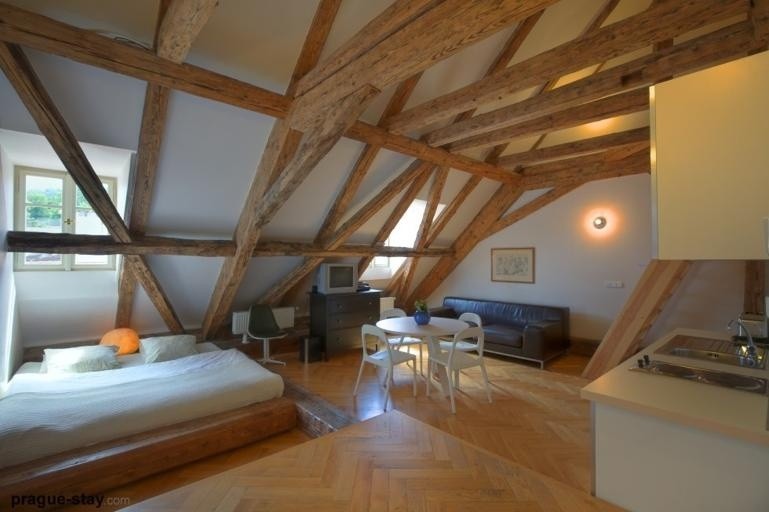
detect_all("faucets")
[725,318,756,349]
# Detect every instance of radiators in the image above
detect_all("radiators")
[232,307,295,334]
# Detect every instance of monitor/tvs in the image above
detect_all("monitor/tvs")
[318,262,358,294]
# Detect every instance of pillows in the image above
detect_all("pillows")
[39,327,198,376]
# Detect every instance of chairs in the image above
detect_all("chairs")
[246,304,288,366]
[353,308,492,415]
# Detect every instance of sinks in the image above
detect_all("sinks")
[669,348,758,370]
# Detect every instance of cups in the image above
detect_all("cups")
[312,285,317,295]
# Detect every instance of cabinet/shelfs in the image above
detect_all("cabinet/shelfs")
[304,289,385,362]
[592,400,768,512]
[649,50,769,259]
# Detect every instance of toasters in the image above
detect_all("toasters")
[739,310,768,339]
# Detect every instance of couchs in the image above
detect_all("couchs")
[430,296,569,370]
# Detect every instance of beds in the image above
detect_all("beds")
[0,342,297,512]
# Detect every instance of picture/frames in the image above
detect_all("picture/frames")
[491,247,535,284]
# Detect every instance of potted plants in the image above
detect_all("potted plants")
[414,299,430,325]
